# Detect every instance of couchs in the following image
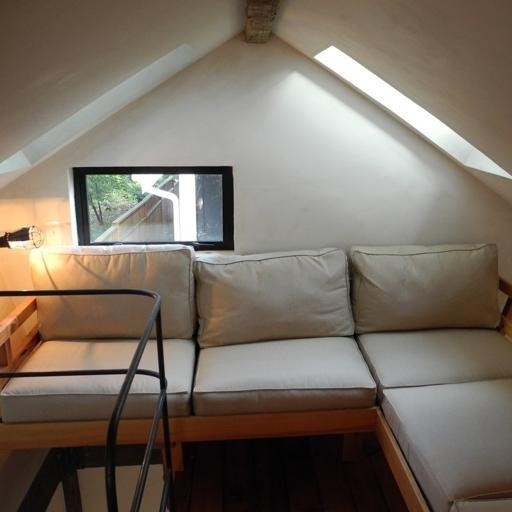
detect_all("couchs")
[0,243,512,511]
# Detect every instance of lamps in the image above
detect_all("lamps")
[0,226,44,252]
